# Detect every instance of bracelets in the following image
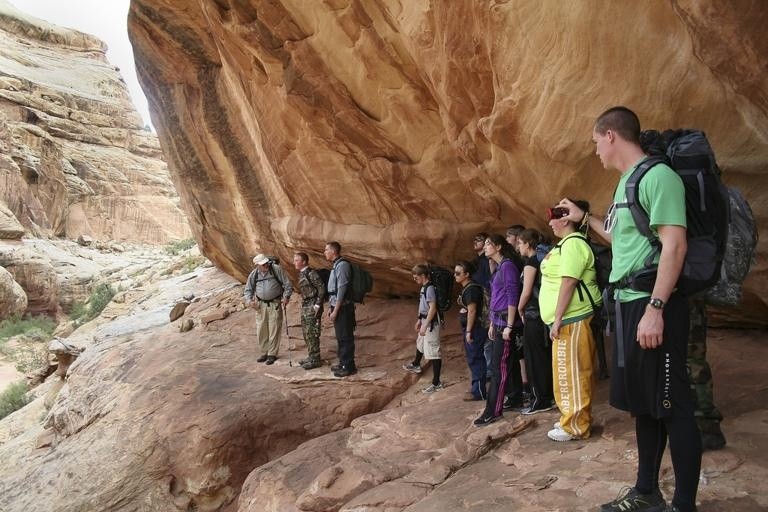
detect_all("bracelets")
[578,211,593,233]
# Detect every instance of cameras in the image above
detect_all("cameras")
[545,208,563,219]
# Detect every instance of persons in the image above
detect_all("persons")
[292,253,325,371]
[324,241,356,377]
[243,254,292,365]
[453,224,725,451]
[537,199,602,443]
[552,105,703,511]
[401,264,441,393]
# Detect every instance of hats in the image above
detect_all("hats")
[253,253,269,265]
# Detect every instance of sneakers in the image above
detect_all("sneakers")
[601,486,666,512]
[257,355,267,362]
[546,427,590,442]
[463,394,482,402]
[474,410,504,427]
[421,381,442,394]
[402,362,422,373]
[266,355,277,365]
[331,364,341,372]
[299,358,310,364]
[502,390,558,414]
[554,421,561,428]
[302,361,321,369]
[334,367,358,377]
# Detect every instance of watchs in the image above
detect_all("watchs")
[645,297,664,309]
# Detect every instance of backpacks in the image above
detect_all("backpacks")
[560,234,613,312]
[334,258,374,304]
[252,255,281,292]
[306,266,333,301]
[422,265,454,311]
[621,127,759,313]
